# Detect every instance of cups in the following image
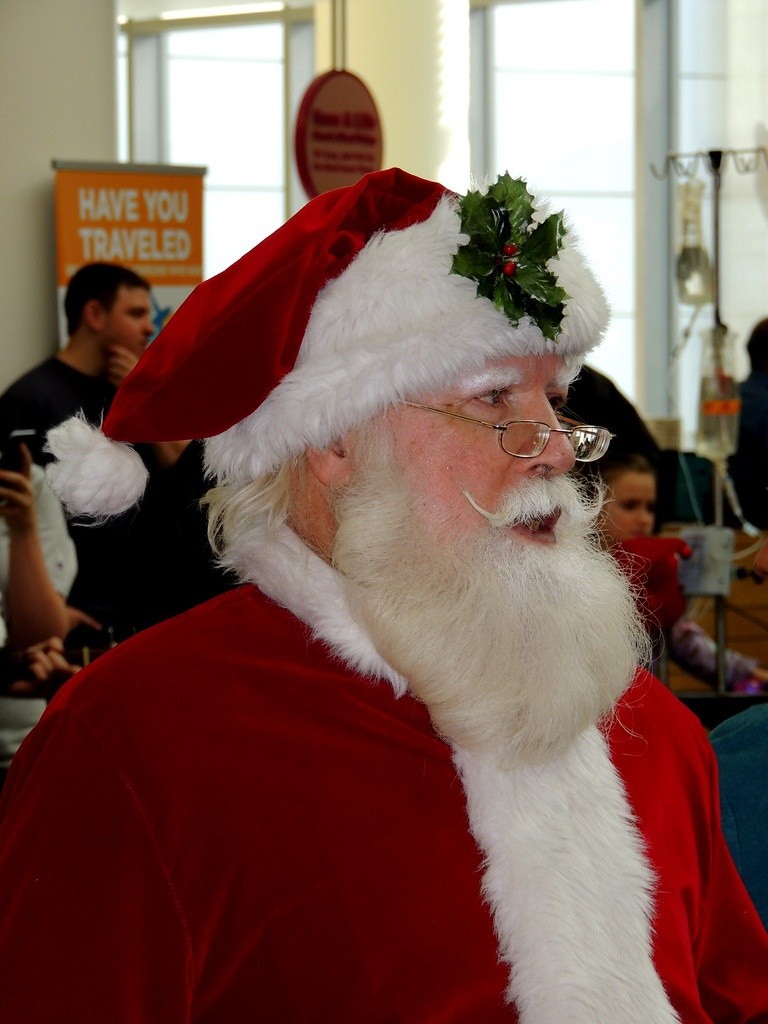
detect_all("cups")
[675,525,735,597]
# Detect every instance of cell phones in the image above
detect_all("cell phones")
[1,428,36,488]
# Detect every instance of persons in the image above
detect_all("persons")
[0,167,768,1024]
[551,317,768,701]
[0,261,240,720]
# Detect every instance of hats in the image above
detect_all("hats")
[45,171,607,516]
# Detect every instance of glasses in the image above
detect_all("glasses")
[393,398,615,464]
